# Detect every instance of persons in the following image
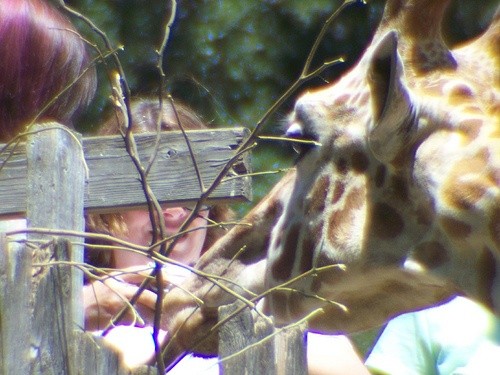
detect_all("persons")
[0,0,169,359]
[82,98,374,374]
[364,295,499,375]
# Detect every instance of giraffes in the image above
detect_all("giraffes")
[159,0,500,358]
